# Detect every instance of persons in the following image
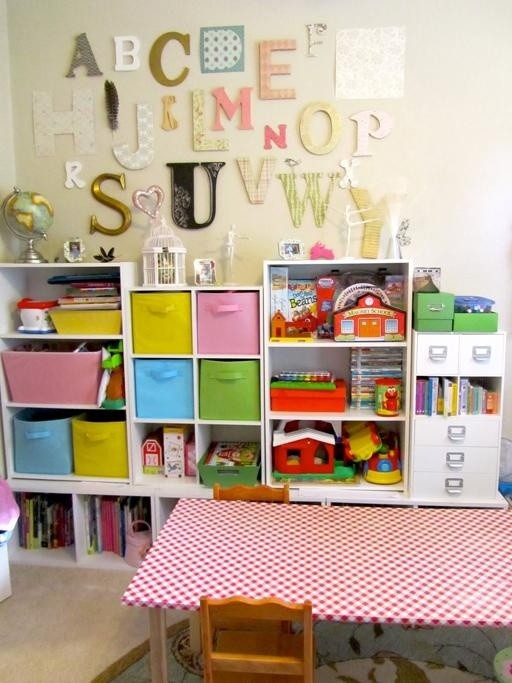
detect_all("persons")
[223,224,251,286]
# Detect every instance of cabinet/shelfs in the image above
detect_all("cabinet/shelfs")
[0,260,509,572]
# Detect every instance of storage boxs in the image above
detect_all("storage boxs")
[133,360,194,419]
[270,379,346,413]
[412,292,453,332]
[17,297,59,332]
[132,292,192,355]
[454,311,497,333]
[71,410,130,478]
[12,408,73,474]
[196,291,260,354]
[197,358,260,421]
[1,340,104,405]
[47,309,121,335]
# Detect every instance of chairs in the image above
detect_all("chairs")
[213,480,290,503]
[198,593,314,682]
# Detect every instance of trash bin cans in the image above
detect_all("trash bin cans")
[0,480,19,603]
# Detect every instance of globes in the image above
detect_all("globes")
[0,186,54,263]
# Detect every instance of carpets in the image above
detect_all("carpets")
[89,617,512,683]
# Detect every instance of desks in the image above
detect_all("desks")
[121,498,511,682]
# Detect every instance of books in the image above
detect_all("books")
[47,273,121,310]
[84,496,149,558]
[15,492,74,549]
[416,378,498,416]
[350,348,403,410]
